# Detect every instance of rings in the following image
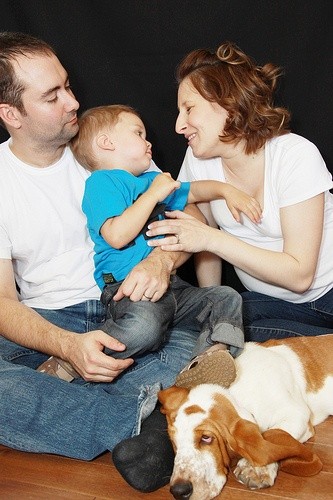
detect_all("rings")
[175,234,181,244]
[143,294,152,300]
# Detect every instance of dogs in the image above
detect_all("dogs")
[156,330,333,500]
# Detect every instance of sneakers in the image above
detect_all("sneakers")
[35,355,80,383]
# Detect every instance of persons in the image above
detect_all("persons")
[0,30,208,493]
[34,103,264,389]
[145,39,332,343]
